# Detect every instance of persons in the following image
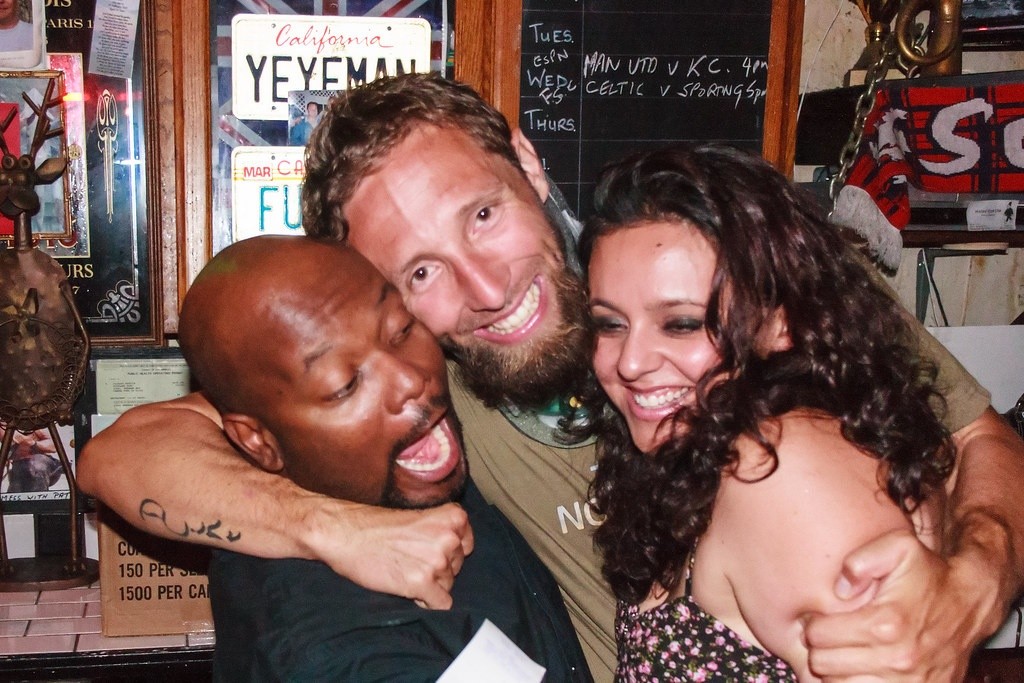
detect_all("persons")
[75,70,1024,682]
[177,234,595,681]
[575,142,957,682]
